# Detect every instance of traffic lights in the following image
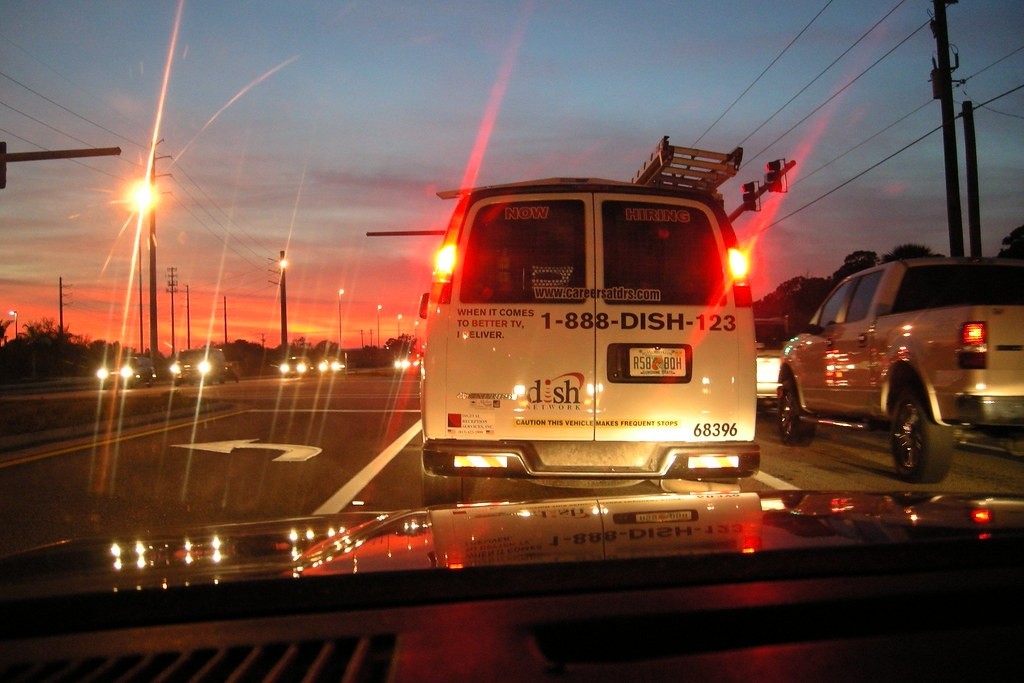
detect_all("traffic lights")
[763,162,783,193]
[740,181,757,210]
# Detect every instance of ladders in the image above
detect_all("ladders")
[631,133,745,193]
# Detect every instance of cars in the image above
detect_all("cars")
[777,256,1024,483]
[753,315,788,420]
[99,356,157,391]
[280,356,313,379]
[171,348,227,387]
[318,357,345,377]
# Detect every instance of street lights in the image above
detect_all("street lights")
[398,314,402,339]
[279,260,289,344]
[338,289,344,348]
[10,311,19,340]
[139,181,150,356]
[414,320,419,342]
[376,305,383,348]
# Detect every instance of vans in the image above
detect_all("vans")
[418,136,758,506]
